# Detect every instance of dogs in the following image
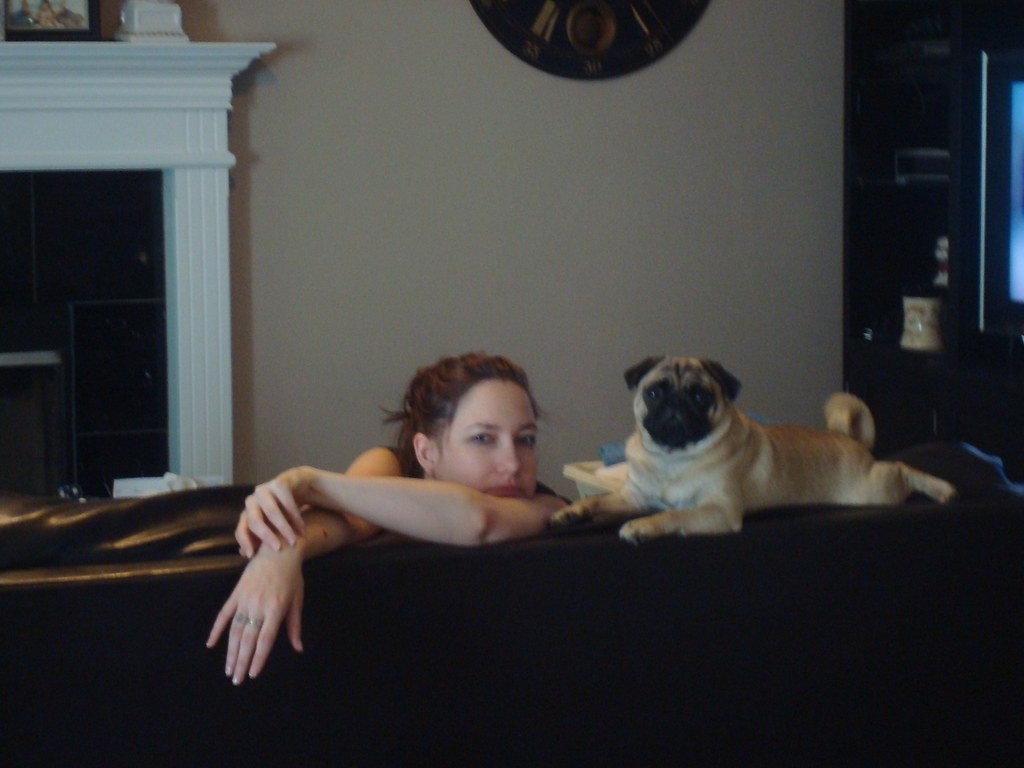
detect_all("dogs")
[547,352,960,546]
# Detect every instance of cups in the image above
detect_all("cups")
[904,297,941,348]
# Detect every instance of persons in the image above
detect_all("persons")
[206,349,574,686]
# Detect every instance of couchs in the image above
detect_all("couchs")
[0,445,1024,768]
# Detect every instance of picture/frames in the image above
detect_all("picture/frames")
[4,0,104,41]
